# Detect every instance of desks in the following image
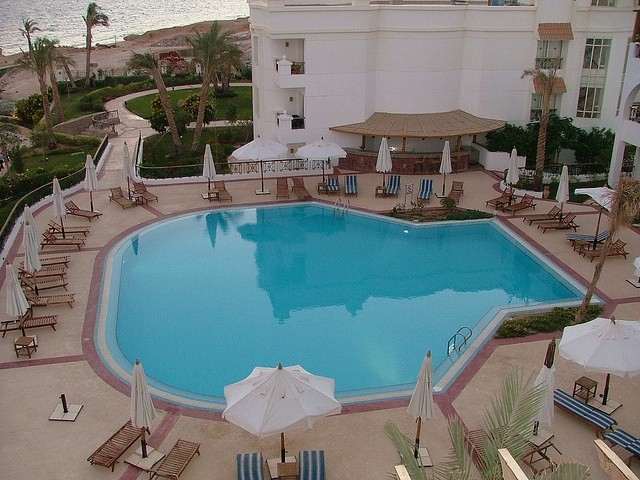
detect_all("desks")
[610,443,634,465]
[374,185,386,199]
[130,193,144,207]
[317,182,328,195]
[573,239,590,254]
[496,197,510,213]
[572,375,598,404]
[207,190,220,202]
[275,462,299,480]
[291,175,312,202]
[14,336,38,359]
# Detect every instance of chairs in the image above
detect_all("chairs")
[385,175,402,199]
[343,175,358,197]
[0,314,59,338]
[447,180,464,204]
[108,185,134,210]
[416,178,433,205]
[64,200,103,223]
[564,229,613,243]
[552,388,615,436]
[491,424,558,479]
[325,177,341,193]
[523,205,561,225]
[19,275,69,292]
[132,181,158,205]
[536,211,580,233]
[39,229,85,251]
[213,179,233,203]
[86,419,151,473]
[583,238,629,262]
[605,428,640,457]
[234,451,264,480]
[21,286,76,309]
[486,187,515,210]
[149,438,201,480]
[17,267,68,281]
[461,428,507,480]
[298,449,327,480]
[503,193,537,216]
[276,176,290,200]
[47,219,91,238]
[20,255,71,269]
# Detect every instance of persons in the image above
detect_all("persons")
[439,140,453,195]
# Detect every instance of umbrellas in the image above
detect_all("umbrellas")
[231,135,289,191]
[6,259,29,337]
[375,136,393,193]
[22,220,42,294]
[558,314,640,405]
[505,145,519,206]
[295,137,347,188]
[129,358,158,458]
[530,336,556,435]
[83,155,98,212]
[574,183,637,249]
[555,163,570,221]
[53,176,67,238]
[406,350,436,458]
[23,203,41,248]
[222,362,343,462]
[123,141,144,199]
[203,144,216,196]
